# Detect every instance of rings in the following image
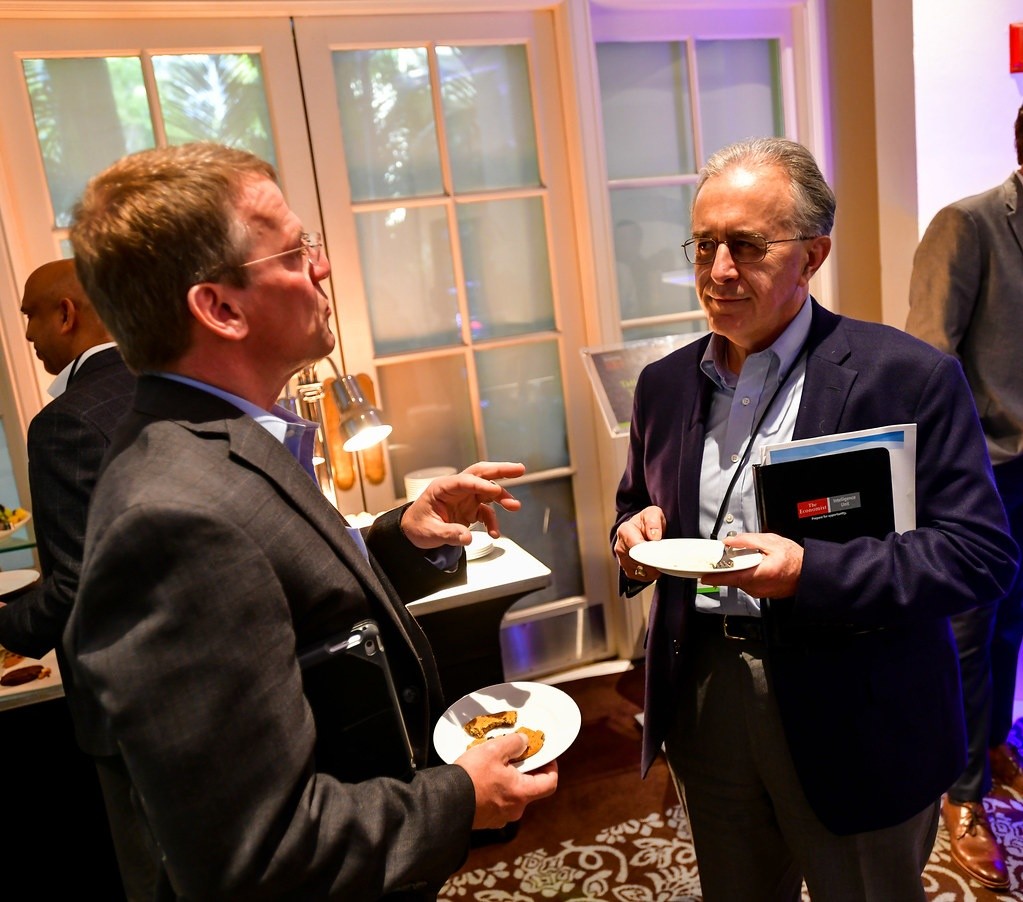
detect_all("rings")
[634,565,648,577]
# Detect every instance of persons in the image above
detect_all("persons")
[609,138,1022,902]
[59,141,560,902]
[906,104,1023,892]
[0,258,141,693]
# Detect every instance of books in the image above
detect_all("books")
[750,421,917,636]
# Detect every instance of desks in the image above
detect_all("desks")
[0,531,553,845]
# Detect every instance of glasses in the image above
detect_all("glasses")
[681,234,814,265]
[212,230,322,280]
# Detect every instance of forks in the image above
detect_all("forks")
[712,532,737,569]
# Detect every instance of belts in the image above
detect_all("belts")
[692,613,761,639]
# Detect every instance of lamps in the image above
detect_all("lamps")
[296,355,396,510]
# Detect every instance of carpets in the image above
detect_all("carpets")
[435,739,1023,902]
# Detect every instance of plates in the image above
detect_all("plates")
[0,569,40,596]
[0,511,32,538]
[433,680,582,773]
[403,466,458,501]
[628,538,763,578]
[465,530,495,561]
